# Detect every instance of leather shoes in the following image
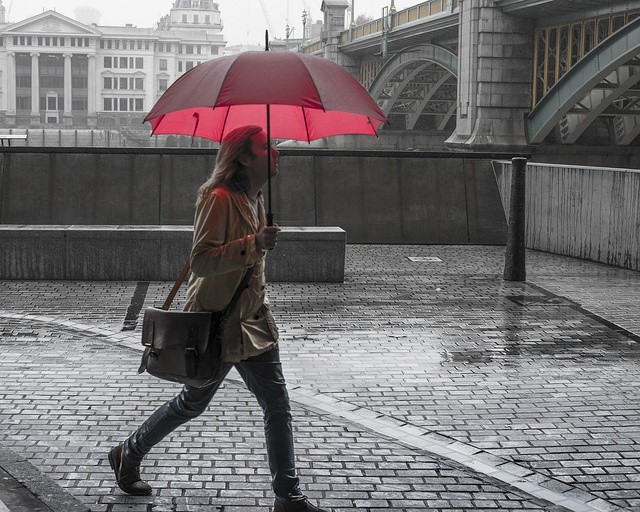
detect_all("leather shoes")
[108,444,152,495]
[274,489,327,512]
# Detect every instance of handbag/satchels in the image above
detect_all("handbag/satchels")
[138,306,224,389]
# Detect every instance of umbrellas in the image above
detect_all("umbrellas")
[142,30,390,252]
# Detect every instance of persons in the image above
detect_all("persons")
[108,125,327,512]
